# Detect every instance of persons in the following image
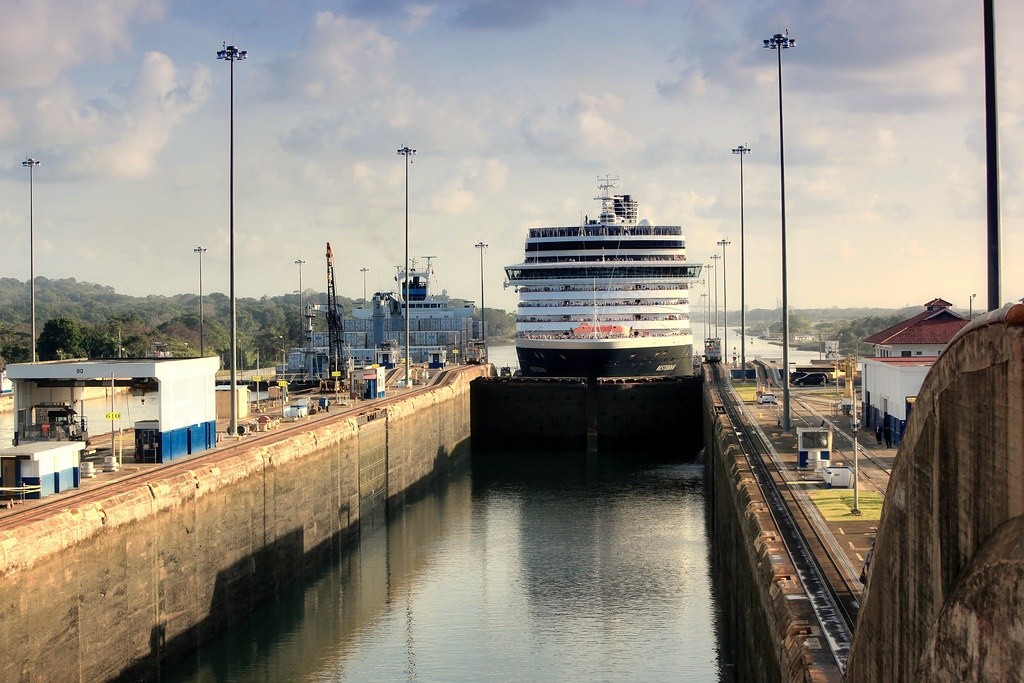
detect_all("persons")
[321,381,326,394]
[525,226,686,262]
[516,285,689,340]
[328,380,334,392]
[884,425,892,449]
[875,424,884,444]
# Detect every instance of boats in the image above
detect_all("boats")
[573,321,631,337]
[351,255,477,321]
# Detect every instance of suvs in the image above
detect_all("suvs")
[792,372,829,388]
[790,372,808,384]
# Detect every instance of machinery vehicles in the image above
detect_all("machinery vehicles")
[320,242,350,393]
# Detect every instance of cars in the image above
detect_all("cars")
[758,393,777,405]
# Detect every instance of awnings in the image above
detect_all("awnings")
[363,374,377,381]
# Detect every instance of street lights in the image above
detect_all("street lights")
[717,239,733,364]
[295,259,307,347]
[836,332,844,395]
[216,39,251,434]
[396,147,418,389]
[279,335,286,376]
[969,294,976,322]
[22,157,41,364]
[238,339,243,384]
[709,254,723,338]
[360,268,369,309]
[730,145,752,384]
[474,242,491,341]
[255,347,260,409]
[701,293,708,340]
[280,349,285,418]
[704,264,714,337]
[193,246,208,358]
[760,29,799,433]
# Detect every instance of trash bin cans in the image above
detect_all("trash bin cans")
[319,396,329,409]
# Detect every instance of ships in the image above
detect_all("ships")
[501,172,707,451]
